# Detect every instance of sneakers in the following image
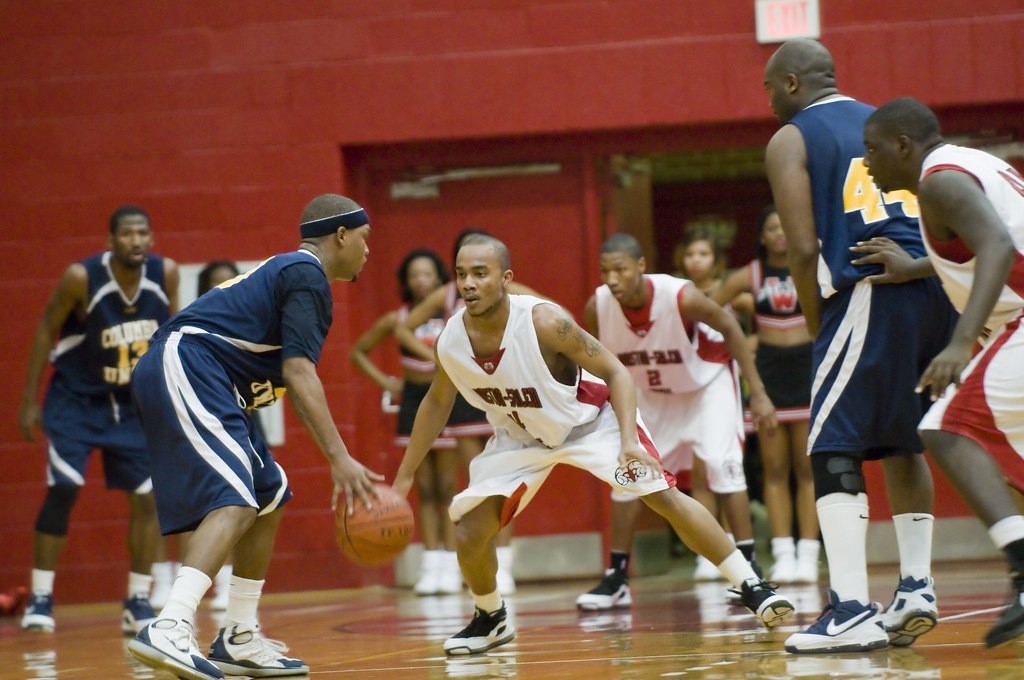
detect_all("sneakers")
[575,568,632,610]
[121,593,158,632]
[128,615,224,680]
[20,593,55,631]
[727,577,794,629]
[985,572,1024,647]
[784,588,888,653]
[442,599,515,656]
[883,571,938,647]
[208,626,309,676]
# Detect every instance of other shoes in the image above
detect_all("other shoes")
[412,550,464,595]
[770,537,821,583]
[495,547,515,593]
[694,556,722,580]
[210,567,232,609]
[148,562,174,607]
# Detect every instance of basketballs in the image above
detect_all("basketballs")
[335,482,415,567]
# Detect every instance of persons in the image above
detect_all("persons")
[127,193,385,680]
[392,232,795,655]
[20,207,179,634]
[149,261,238,610]
[764,38,962,653]
[347,200,820,595]
[848,98,1024,648]
